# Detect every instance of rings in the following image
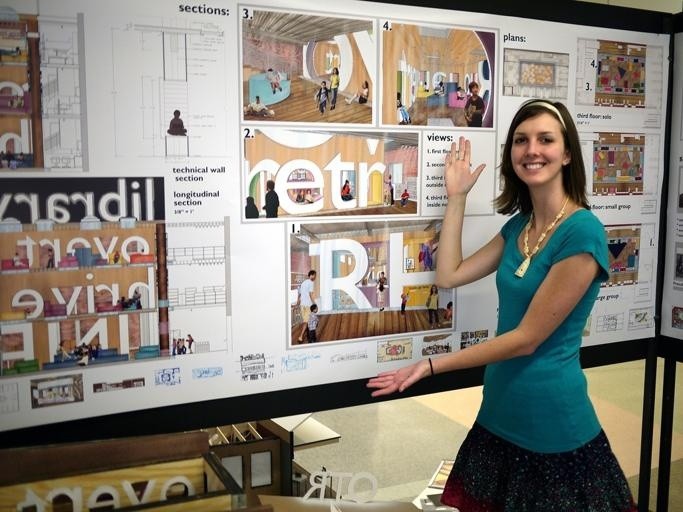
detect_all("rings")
[459,158,464,160]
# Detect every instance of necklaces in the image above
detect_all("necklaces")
[514,196,569,278]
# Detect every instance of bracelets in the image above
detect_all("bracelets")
[428,358,434,376]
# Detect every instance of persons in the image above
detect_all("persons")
[401,288,408,315]
[435,85,444,97]
[341,179,353,201]
[266,68,282,94]
[308,304,319,343]
[167,110,186,136]
[13,248,193,360]
[384,175,395,206]
[297,270,316,342]
[317,81,329,118]
[421,238,433,271]
[444,302,453,321]
[377,272,387,311]
[366,99,632,512]
[424,81,429,92]
[329,68,340,110]
[426,284,441,329]
[0,47,25,167]
[464,82,484,127]
[401,189,410,207]
[296,189,305,202]
[457,87,464,100]
[245,197,259,218]
[263,180,279,218]
[304,189,314,203]
[345,81,368,105]
[397,92,411,125]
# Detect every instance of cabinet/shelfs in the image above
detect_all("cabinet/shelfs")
[291,271,308,286]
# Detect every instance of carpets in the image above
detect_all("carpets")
[427,117,454,127]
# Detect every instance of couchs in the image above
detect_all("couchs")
[0,249,159,376]
[418,83,467,108]
[249,72,291,107]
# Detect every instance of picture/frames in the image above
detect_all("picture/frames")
[406,258,415,269]
